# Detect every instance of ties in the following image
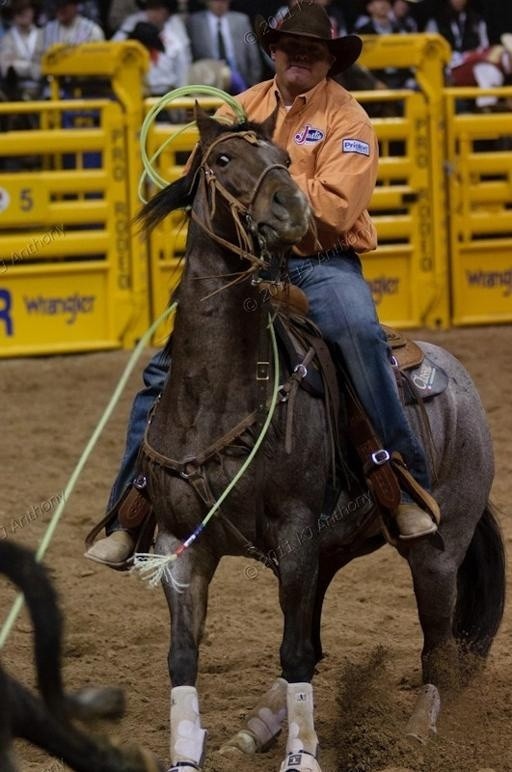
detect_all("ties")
[217,21,226,60]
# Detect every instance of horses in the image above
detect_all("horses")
[126,97,506,772]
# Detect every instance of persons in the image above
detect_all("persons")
[80,1,439,569]
[0,1,511,172]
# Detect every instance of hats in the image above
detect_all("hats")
[122,22,166,55]
[254,0,362,77]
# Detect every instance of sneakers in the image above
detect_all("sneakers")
[83,530,133,567]
[391,490,439,541]
